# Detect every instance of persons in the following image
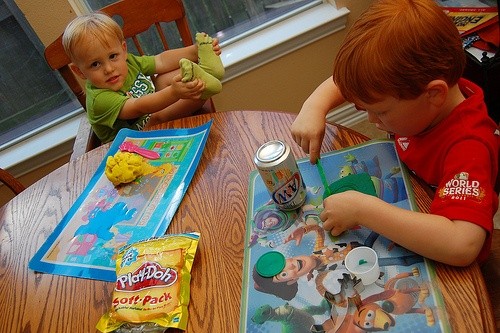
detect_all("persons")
[290,1,499,267]
[62,10,225,143]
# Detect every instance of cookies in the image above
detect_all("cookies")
[94,230,200,333]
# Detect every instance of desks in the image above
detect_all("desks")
[0,108,495,333]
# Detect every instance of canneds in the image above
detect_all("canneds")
[253,139,307,211]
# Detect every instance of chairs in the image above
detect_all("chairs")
[43,0,219,163]
